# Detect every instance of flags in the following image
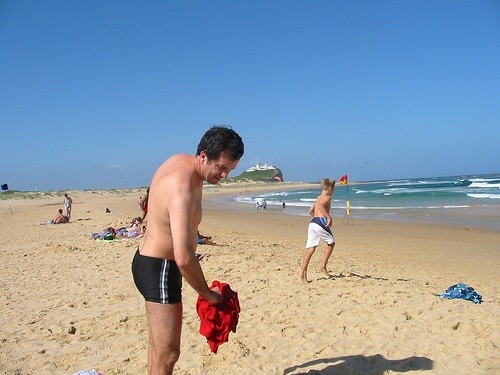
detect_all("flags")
[338,174,348,186]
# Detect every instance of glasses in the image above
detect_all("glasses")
[210,156,229,174]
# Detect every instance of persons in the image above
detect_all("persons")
[88,186,150,241]
[131,124,244,375]
[298,177,337,284]
[256,199,286,212]
[50,193,73,225]
